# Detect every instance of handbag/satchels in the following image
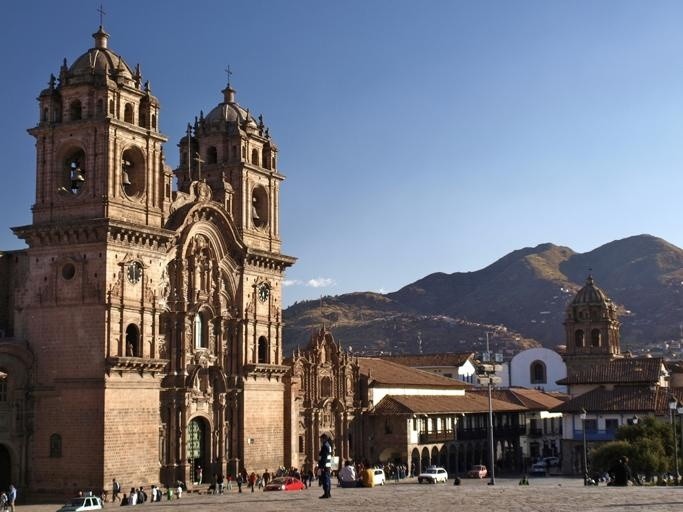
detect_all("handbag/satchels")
[356,479,364,488]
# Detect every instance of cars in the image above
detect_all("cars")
[263,477,304,492]
[56,497,101,512]
[372,469,385,486]
[528,456,560,477]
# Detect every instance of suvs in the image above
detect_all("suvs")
[418,468,447,484]
[467,465,487,479]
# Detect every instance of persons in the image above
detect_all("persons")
[208,471,262,495]
[318,434,331,498]
[119,486,147,506]
[196,465,203,485]
[276,465,321,488]
[114,478,120,502]
[336,462,407,487]
[262,469,270,486]
[0,493,8,512]
[8,485,17,512]
[151,484,183,502]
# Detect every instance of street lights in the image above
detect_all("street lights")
[632,413,638,425]
[667,393,680,485]
[578,408,588,486]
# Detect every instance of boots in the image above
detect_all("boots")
[318,489,330,499]
